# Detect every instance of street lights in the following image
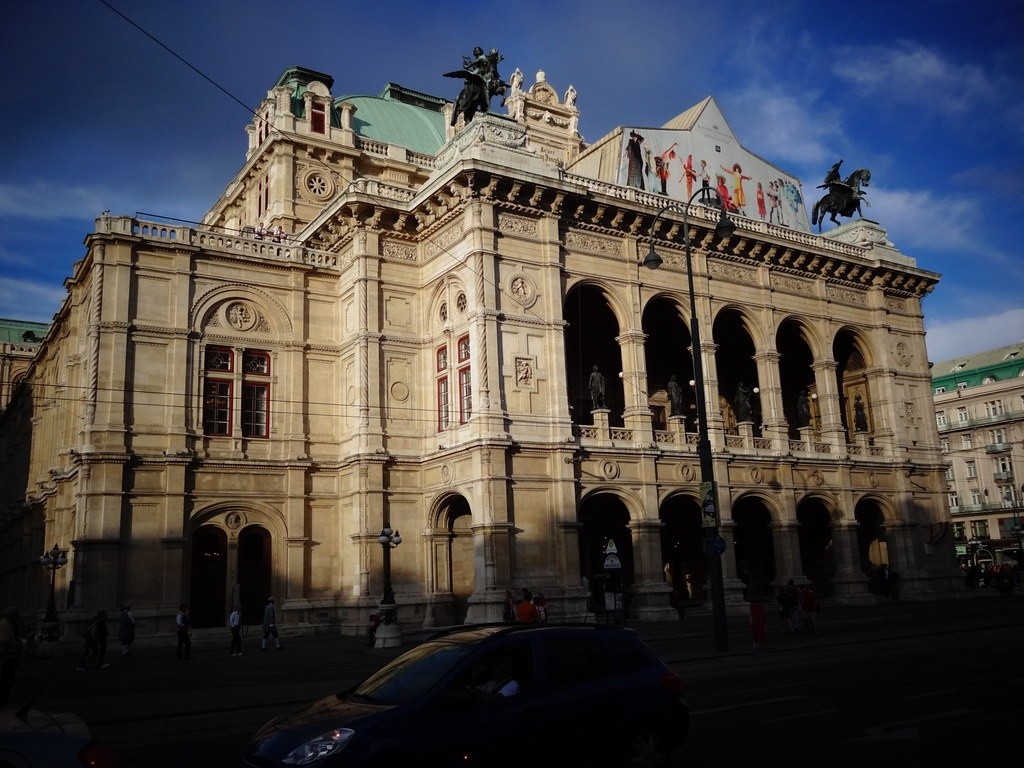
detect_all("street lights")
[372,524,403,648]
[37,541,69,642]
[642,186,739,653]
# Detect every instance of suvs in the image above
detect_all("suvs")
[240,617,694,768]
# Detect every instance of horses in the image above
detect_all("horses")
[811,168,871,233]
[450,48,508,127]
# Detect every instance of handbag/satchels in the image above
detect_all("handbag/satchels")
[177,615,189,628]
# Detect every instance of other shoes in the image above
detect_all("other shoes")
[101,664,111,668]
[261,646,267,650]
[122,651,131,656]
[681,615,690,619]
[177,654,192,661]
[277,646,282,650]
[231,652,244,658]
[77,666,86,670]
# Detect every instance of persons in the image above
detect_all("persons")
[589,366,608,409]
[254,223,268,239]
[733,383,754,422]
[230,606,243,656]
[776,578,820,636]
[510,68,524,93]
[176,604,193,661]
[464,47,492,112]
[76,610,112,671]
[565,84,577,106]
[0,606,39,710]
[854,395,869,431]
[536,68,546,82]
[668,375,684,416]
[503,588,548,625]
[118,605,136,656]
[272,226,287,243]
[262,596,282,651]
[823,159,852,195]
[749,591,769,649]
[958,560,1019,588]
[797,391,813,427]
[663,560,696,620]
